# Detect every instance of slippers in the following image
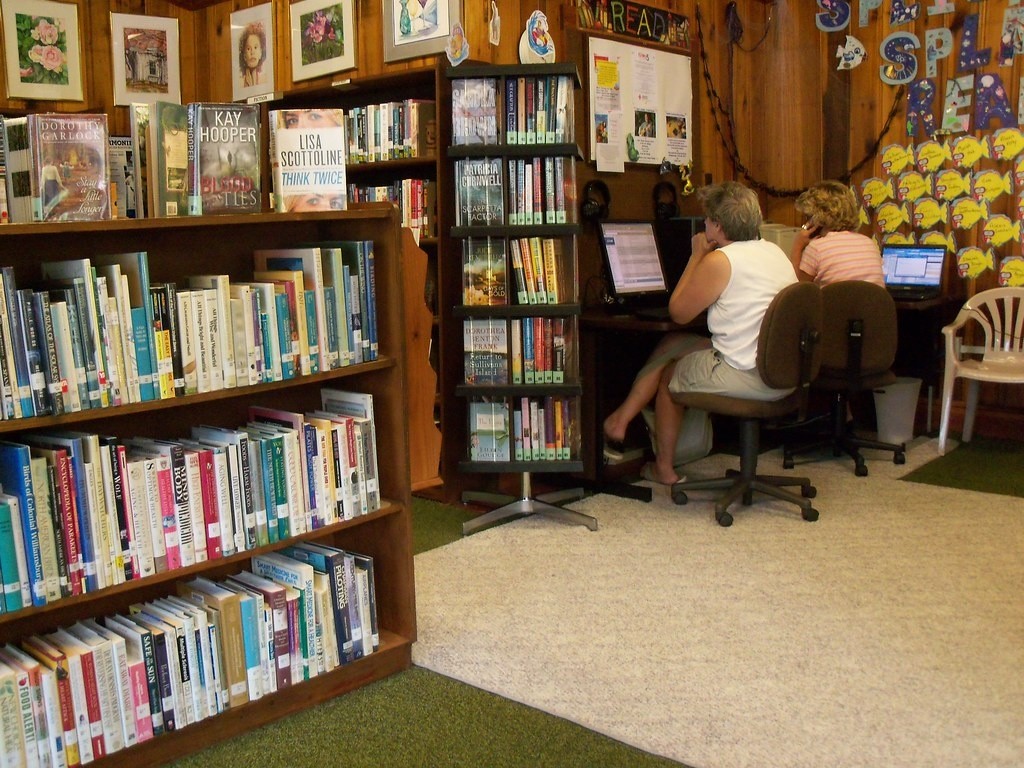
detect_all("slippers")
[601,411,629,448]
[640,460,687,486]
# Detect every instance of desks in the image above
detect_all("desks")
[583,308,677,498]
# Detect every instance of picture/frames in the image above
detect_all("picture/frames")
[1,1,84,103]
[289,0,358,83]
[110,12,182,106]
[231,1,279,102]
[381,0,454,64]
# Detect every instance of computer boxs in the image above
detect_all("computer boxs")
[652,215,708,299]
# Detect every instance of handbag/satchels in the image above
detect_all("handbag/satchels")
[643,406,713,466]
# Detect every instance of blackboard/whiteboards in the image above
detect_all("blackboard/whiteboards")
[585,32,698,170]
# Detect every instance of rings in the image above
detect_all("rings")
[801,225,807,230]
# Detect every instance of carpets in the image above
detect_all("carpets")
[410,454,1024,768]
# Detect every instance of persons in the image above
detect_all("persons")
[600,180,799,487]
[637,113,655,138]
[282,195,346,212]
[41,156,66,208]
[274,109,343,130]
[759,179,885,435]
[237,21,269,88]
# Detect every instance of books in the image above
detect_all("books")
[0,390,381,768]
[0,240,378,422]
[450,75,580,461]
[0,99,437,248]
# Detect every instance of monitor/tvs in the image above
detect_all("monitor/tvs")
[596,215,669,312]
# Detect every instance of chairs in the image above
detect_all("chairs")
[669,283,819,525]
[937,286,1024,455]
[783,279,906,478]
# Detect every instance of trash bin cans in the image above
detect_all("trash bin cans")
[871,376,922,444]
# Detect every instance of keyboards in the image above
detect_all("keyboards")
[634,305,671,323]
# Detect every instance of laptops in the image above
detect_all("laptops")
[878,243,947,301]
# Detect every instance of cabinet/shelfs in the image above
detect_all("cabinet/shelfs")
[304,70,444,408]
[0,205,419,768]
[448,66,599,537]
